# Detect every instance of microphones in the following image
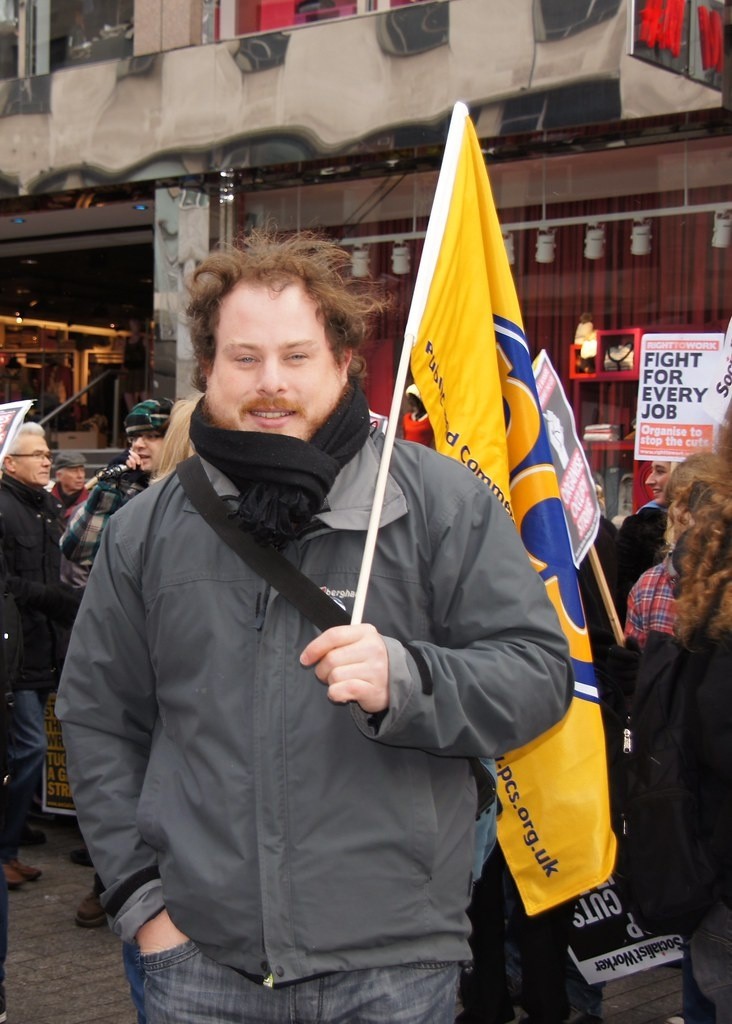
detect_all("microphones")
[97,461,141,482]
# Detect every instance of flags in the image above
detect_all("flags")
[411,115,618,917]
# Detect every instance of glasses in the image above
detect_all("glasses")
[127,430,163,443]
[9,451,54,464]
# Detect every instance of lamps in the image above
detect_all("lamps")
[349,246,369,280]
[583,226,605,258]
[391,245,410,274]
[712,212,732,248]
[502,234,514,264]
[534,230,556,263]
[631,223,653,255]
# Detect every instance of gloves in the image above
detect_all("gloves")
[604,634,643,695]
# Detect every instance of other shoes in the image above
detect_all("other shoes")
[71,849,93,866]
[75,873,108,927]
[8,857,41,880]
[16,826,47,846]
[2,863,25,885]
[0,984,8,1024]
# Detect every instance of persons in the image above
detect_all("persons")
[0,394,201,1024]
[402,385,732,1024]
[51,224,574,1024]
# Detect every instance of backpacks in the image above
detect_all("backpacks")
[589,628,721,942]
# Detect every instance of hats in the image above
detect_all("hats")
[54,449,87,467]
[125,397,174,433]
[406,384,423,404]
